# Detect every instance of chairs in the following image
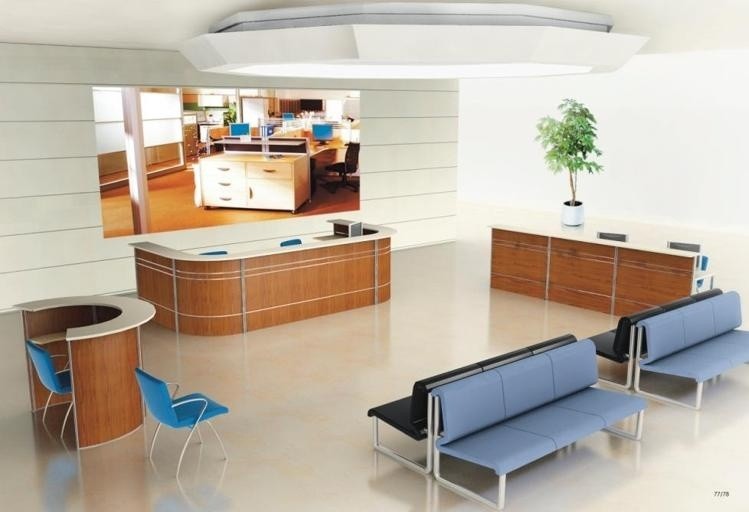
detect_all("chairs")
[667,241,709,294]
[597,231,628,244]
[586,290,749,411]
[26,341,73,439]
[135,368,229,479]
[280,239,302,247]
[198,251,228,255]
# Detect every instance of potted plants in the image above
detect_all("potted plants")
[535,97,605,228]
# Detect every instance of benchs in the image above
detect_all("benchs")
[368,333,647,512]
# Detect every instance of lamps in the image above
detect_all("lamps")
[177,0,646,78]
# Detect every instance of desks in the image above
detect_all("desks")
[192,117,360,214]
[490,224,720,317]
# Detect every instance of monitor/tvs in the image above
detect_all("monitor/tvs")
[312,124,333,146]
[228,123,250,136]
[282,113,295,119]
[667,241,703,269]
[300,100,323,112]
[597,231,629,242]
